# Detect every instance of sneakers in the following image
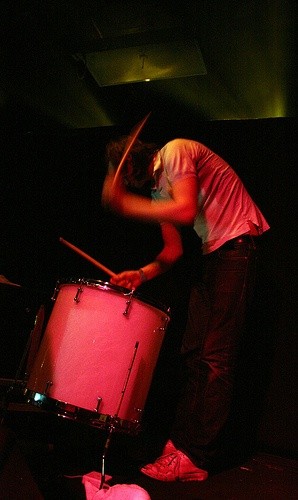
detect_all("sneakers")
[141,450,208,481]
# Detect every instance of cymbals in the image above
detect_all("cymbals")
[0,274,21,288]
[27,304,45,380]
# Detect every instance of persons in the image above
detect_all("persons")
[97,126,271,480]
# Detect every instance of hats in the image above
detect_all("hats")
[106,136,157,164]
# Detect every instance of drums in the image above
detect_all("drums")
[24,275,174,437]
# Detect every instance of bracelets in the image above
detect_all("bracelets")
[136,266,150,285]
